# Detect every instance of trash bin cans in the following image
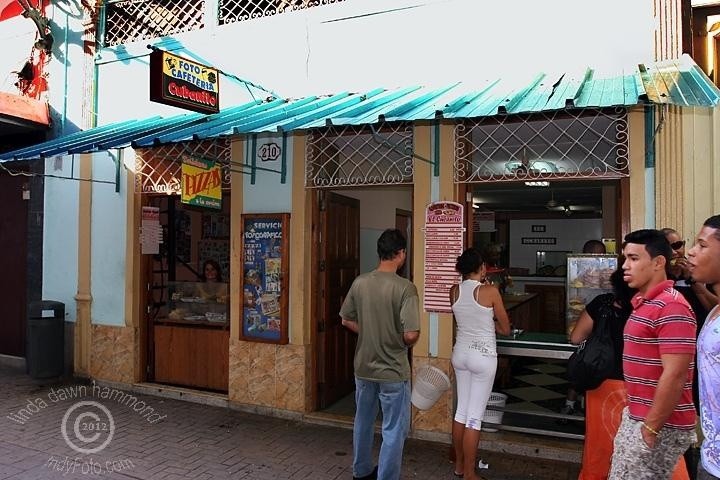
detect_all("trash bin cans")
[26,300,65,379]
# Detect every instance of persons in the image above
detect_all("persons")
[338,227,422,479]
[581,239,607,254]
[447,247,510,479]
[569,267,691,479]
[557,377,585,415]
[605,229,699,480]
[172,259,226,298]
[660,227,720,480]
[686,214,720,480]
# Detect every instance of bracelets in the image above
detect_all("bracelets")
[643,423,659,435]
[685,276,696,286]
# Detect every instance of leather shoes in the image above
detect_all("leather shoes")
[352,465,378,480]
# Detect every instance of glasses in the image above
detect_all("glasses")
[670,241,683,250]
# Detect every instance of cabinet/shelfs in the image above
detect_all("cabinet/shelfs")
[153,282,230,391]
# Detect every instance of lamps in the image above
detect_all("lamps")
[511,157,551,187]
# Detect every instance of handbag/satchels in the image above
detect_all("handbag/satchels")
[570,294,617,376]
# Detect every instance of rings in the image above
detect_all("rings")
[685,263,687,266]
